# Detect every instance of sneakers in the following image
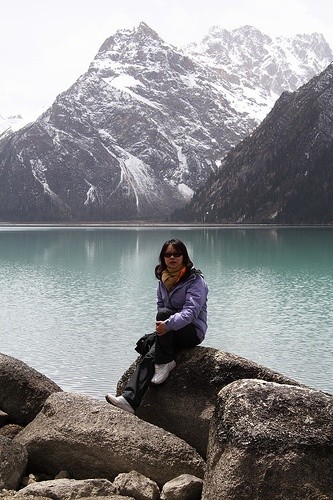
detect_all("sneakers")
[105,393,135,414]
[151,360,176,384]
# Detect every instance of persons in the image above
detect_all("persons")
[105,238,210,415]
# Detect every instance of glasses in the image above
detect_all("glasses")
[163,253,183,258]
